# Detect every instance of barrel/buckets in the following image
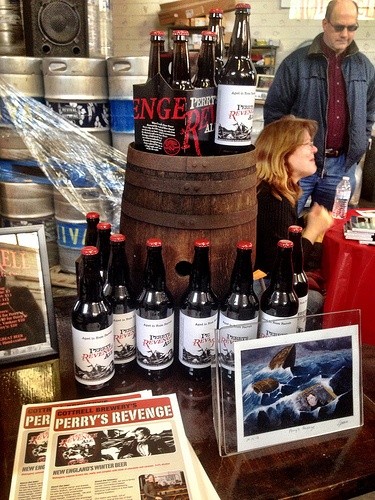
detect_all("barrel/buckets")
[116,142,258,297]
[0,0,148,277]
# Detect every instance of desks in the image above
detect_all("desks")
[313,208,374,348]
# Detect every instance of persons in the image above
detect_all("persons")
[264,0,375,217]
[143,474,162,500]
[255,115,335,333]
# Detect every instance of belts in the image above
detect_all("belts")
[326,149,346,158]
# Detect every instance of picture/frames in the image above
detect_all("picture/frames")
[0,225,60,368]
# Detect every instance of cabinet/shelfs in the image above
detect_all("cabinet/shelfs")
[225,44,279,76]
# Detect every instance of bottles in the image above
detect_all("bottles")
[215,3,258,150]
[70,247,115,390]
[94,222,111,286]
[136,237,177,377]
[102,233,136,370]
[288,224,309,332]
[207,8,223,86]
[146,30,171,87]
[218,239,259,383]
[331,175,350,219]
[192,31,218,91]
[171,29,192,91]
[177,239,219,374]
[258,240,299,338]
[85,211,100,246]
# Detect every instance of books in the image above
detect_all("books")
[343,216,375,245]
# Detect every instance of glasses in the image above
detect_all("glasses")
[301,141,314,146]
[328,19,359,32]
